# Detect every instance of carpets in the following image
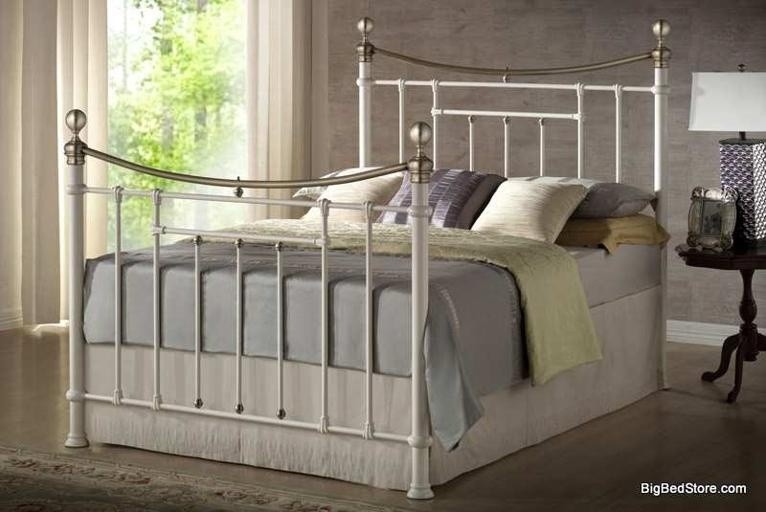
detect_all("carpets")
[0,445,412,512]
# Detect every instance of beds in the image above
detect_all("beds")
[64,17,669,500]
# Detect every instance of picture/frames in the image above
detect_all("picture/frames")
[686,187,740,251]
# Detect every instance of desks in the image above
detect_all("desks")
[676,245,766,404]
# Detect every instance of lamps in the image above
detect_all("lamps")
[688,64,766,248]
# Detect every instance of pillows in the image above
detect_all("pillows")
[299,168,405,222]
[375,170,508,230]
[556,214,670,253]
[471,179,589,242]
[292,171,339,200]
[510,177,657,218]
